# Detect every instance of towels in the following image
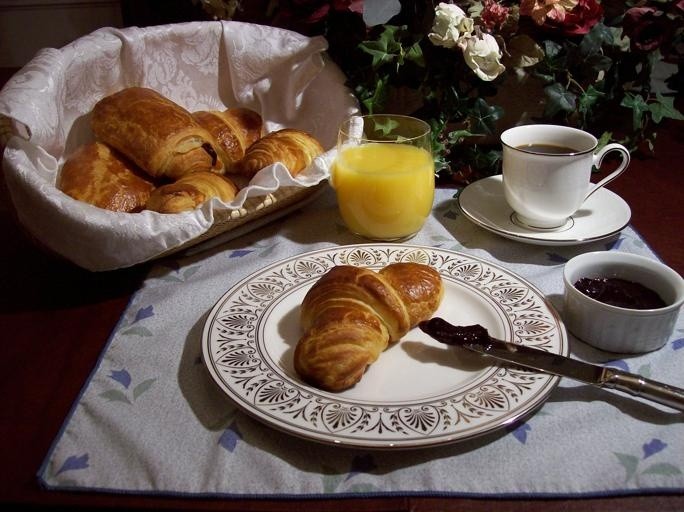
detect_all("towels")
[39,189,683,497]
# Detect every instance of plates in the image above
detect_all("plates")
[455,172,634,247]
[198,241,571,453]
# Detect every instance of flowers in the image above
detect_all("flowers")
[356,0,684,185]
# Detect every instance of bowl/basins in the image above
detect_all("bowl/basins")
[559,248,684,355]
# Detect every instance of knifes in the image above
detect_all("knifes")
[417,315,683,418]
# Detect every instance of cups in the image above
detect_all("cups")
[498,121,631,230]
[331,112,435,244]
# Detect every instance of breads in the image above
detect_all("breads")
[59,86,325,214]
[293,261,444,393]
